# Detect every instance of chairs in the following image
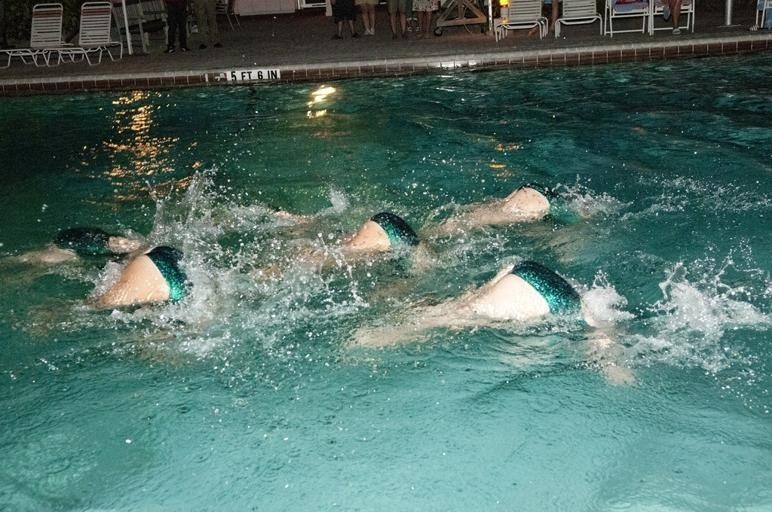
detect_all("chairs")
[604,0,652,38]
[0,2,75,69]
[217,4,243,31]
[493,0,549,42]
[756,1,772,28]
[650,0,696,36]
[554,0,603,39]
[42,1,123,68]
[137,1,192,38]
[113,4,169,54]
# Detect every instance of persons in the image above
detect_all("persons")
[530,0,560,35]
[412,0,438,40]
[388,0,410,40]
[325,0,357,39]
[347,259,631,379]
[435,182,609,239]
[165,1,189,52]
[34,227,142,265]
[2,227,193,306]
[195,0,222,49]
[663,0,682,35]
[355,0,378,37]
[281,212,422,269]
